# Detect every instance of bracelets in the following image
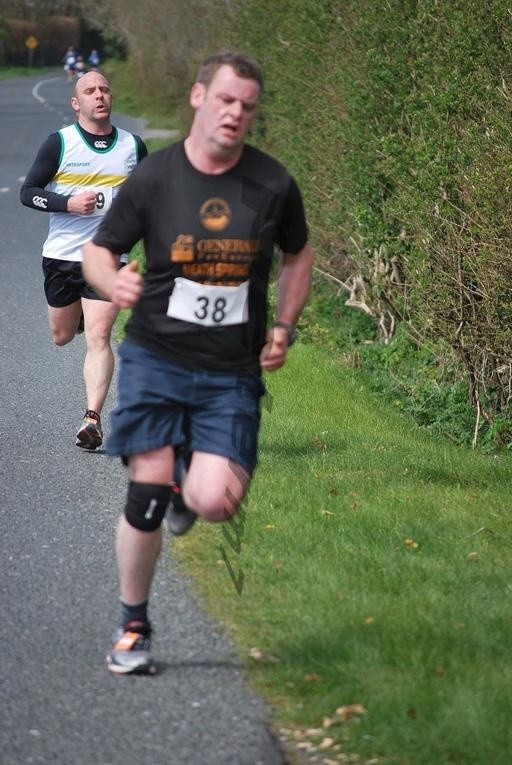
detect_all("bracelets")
[272,320,297,346]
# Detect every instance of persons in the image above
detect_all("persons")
[89,49,99,72]
[73,55,86,79]
[18,70,148,451]
[79,53,315,679]
[62,44,76,85]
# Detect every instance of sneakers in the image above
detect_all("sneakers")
[103,617,157,676]
[165,501,195,537]
[73,412,107,451]
